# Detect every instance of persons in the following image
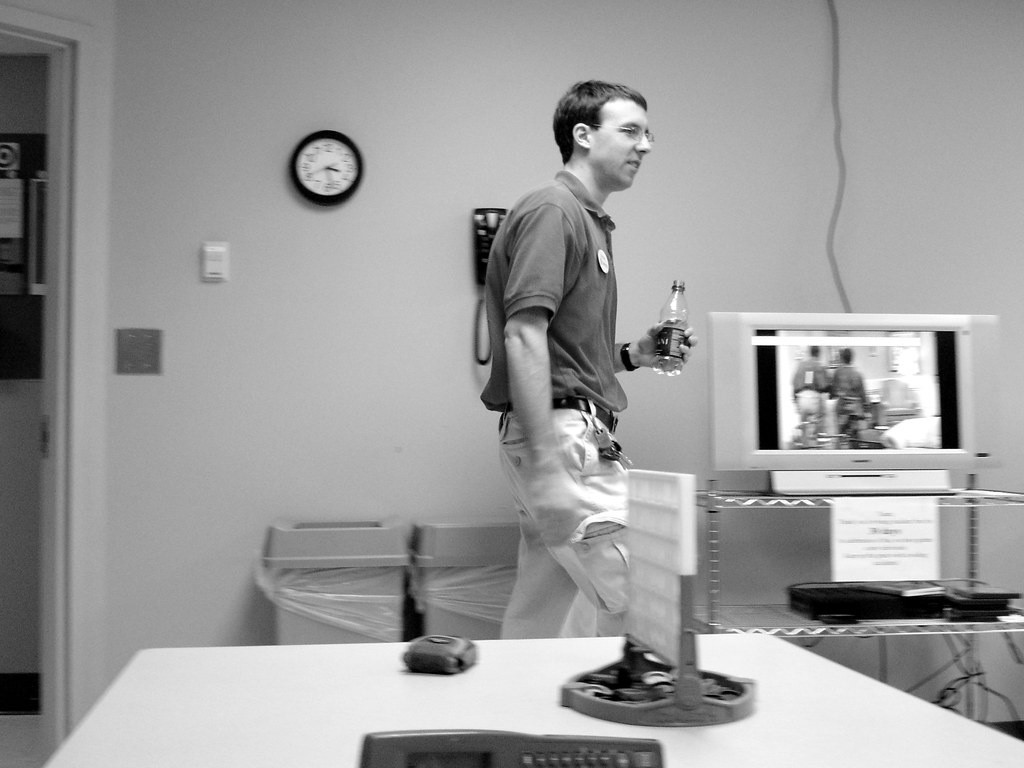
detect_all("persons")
[830,348,866,434]
[479,80,699,639]
[793,346,832,435]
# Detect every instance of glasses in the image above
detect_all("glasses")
[589,125,653,142]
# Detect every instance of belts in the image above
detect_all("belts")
[504,397,618,435]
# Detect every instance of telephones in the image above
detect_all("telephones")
[470,207,513,286]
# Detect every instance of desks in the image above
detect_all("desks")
[43,631,1024,767]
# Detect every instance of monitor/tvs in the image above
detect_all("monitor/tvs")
[706,311,1003,495]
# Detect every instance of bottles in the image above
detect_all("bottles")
[652,279,689,376]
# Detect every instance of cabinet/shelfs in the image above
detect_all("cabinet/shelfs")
[691,487,1024,637]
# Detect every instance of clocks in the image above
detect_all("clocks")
[288,129,362,206]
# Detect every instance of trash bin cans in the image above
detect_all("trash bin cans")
[411,522,523,640]
[257,517,417,647]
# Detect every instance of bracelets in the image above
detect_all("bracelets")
[621,343,638,372]
